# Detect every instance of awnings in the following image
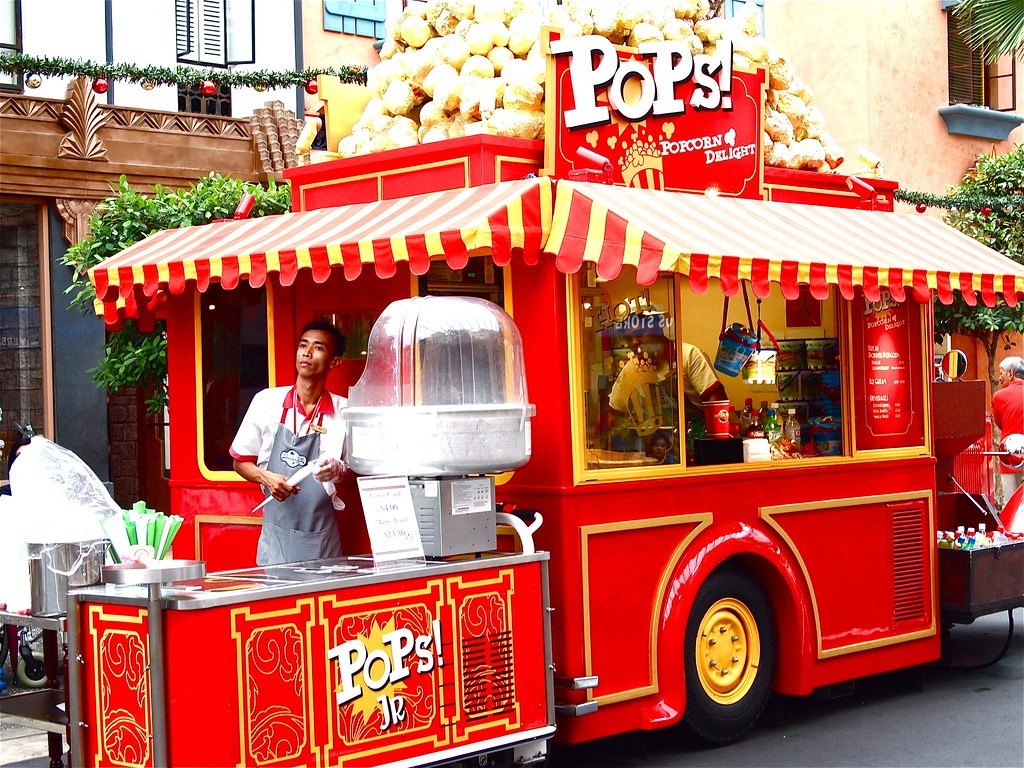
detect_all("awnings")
[544,181,1024,307]
[88,177,552,299]
[94,298,166,332]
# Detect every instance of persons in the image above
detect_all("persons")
[648,434,675,464]
[590,311,739,447]
[993,356,1024,504]
[230,321,351,566]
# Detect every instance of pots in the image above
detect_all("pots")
[25,539,111,618]
[100,560,207,585]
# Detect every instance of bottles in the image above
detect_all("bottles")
[937,522,1024,550]
[729,398,801,450]
[688,418,702,464]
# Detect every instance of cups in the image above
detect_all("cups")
[806,340,837,370]
[701,400,730,439]
[713,322,758,377]
[814,432,841,455]
[776,341,804,370]
